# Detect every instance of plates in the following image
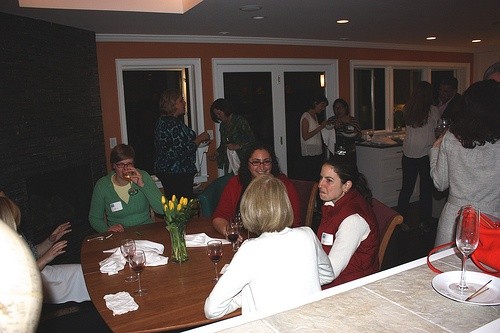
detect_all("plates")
[432,271,500,305]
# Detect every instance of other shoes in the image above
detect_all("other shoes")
[423,221,431,233]
[402,215,409,224]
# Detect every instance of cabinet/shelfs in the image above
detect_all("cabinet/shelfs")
[380,147,421,206]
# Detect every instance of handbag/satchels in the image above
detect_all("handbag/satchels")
[426,204,500,279]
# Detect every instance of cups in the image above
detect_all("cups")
[437,119,448,135]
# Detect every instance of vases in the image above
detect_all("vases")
[169,222,189,262]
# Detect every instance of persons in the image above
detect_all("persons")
[153,90,215,202]
[212,141,302,240]
[0,190,92,303]
[299,95,329,182]
[327,98,362,151]
[429,79,500,254]
[210,97,254,176]
[438,77,463,116]
[484,61,500,83]
[317,156,381,290]
[397,80,443,231]
[204,174,335,319]
[88,143,167,232]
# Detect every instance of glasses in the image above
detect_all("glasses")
[249,160,273,166]
[116,161,134,168]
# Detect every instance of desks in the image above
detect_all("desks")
[81,216,245,333]
[182,248,500,333]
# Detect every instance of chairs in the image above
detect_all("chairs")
[198,175,404,267]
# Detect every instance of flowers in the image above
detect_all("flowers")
[161,193,194,226]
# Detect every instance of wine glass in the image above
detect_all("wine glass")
[206,245,224,283]
[226,213,244,253]
[369,129,374,141]
[120,238,138,283]
[122,168,139,195]
[448,206,481,294]
[130,250,149,296]
